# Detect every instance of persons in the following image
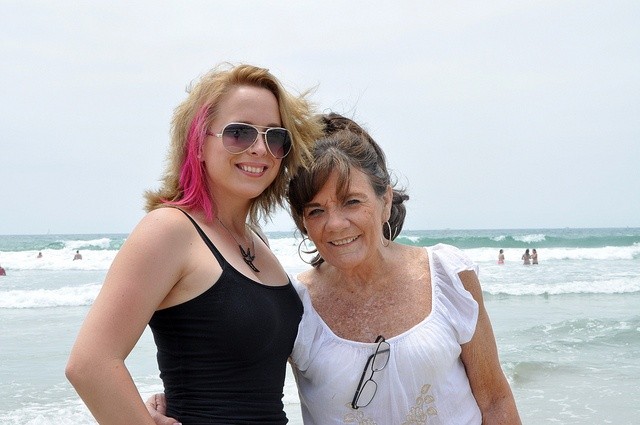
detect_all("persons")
[145,111,523,425]
[498,249,505,264]
[531,249,538,264]
[73,250,82,261]
[38,252,43,258]
[522,249,531,264]
[0,266,6,275]
[65,61,326,425]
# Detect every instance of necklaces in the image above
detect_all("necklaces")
[215,216,259,273]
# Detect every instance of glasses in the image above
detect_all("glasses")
[351,336,391,410]
[206,121,292,158]
